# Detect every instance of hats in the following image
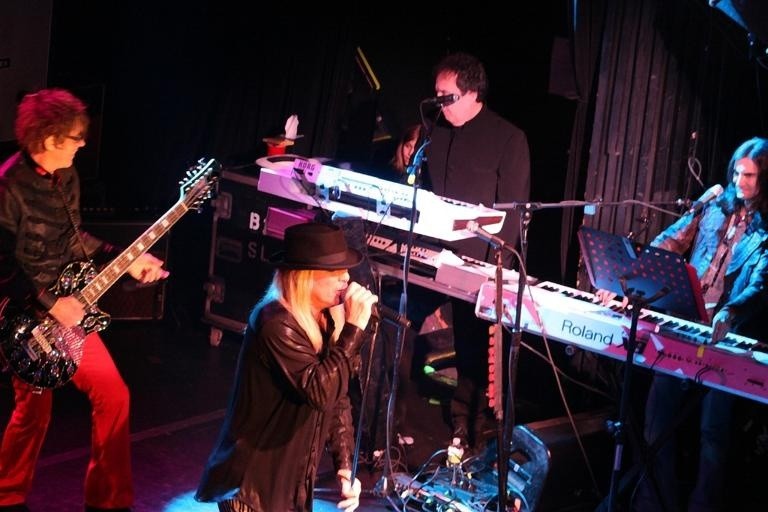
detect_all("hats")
[260,221,364,270]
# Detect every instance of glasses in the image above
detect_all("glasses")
[64,133,83,143]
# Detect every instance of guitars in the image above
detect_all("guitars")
[0,156,224,395]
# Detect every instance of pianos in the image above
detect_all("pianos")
[262,201,538,305]
[475,281,768,406]
[256,154,507,242]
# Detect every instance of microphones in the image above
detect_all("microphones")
[466,220,506,249]
[340,287,411,328]
[686,183,724,216]
[424,94,459,104]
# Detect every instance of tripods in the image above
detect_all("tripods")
[312,105,445,512]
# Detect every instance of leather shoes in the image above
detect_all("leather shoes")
[453,425,475,452]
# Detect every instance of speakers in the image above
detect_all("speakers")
[465,405,644,512]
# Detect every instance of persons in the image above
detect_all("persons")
[385,55,530,449]
[596,138,768,511]
[194,221,378,511]
[0,88,169,512]
